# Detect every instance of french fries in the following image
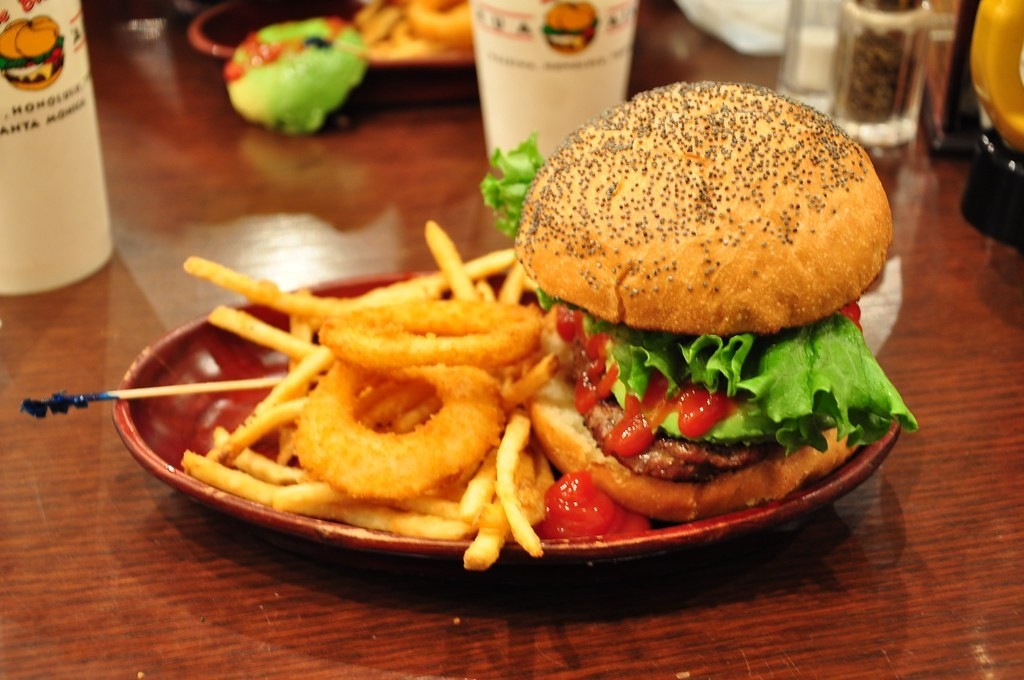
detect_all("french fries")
[180,219,558,570]
[348,0,462,69]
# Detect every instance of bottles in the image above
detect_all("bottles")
[835,0,931,144]
[778,0,837,117]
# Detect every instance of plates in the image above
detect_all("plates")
[187,1,648,103]
[109,269,898,568]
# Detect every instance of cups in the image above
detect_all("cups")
[468,0,640,172]
[0,0,112,297]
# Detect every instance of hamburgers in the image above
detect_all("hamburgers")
[478,81,919,519]
